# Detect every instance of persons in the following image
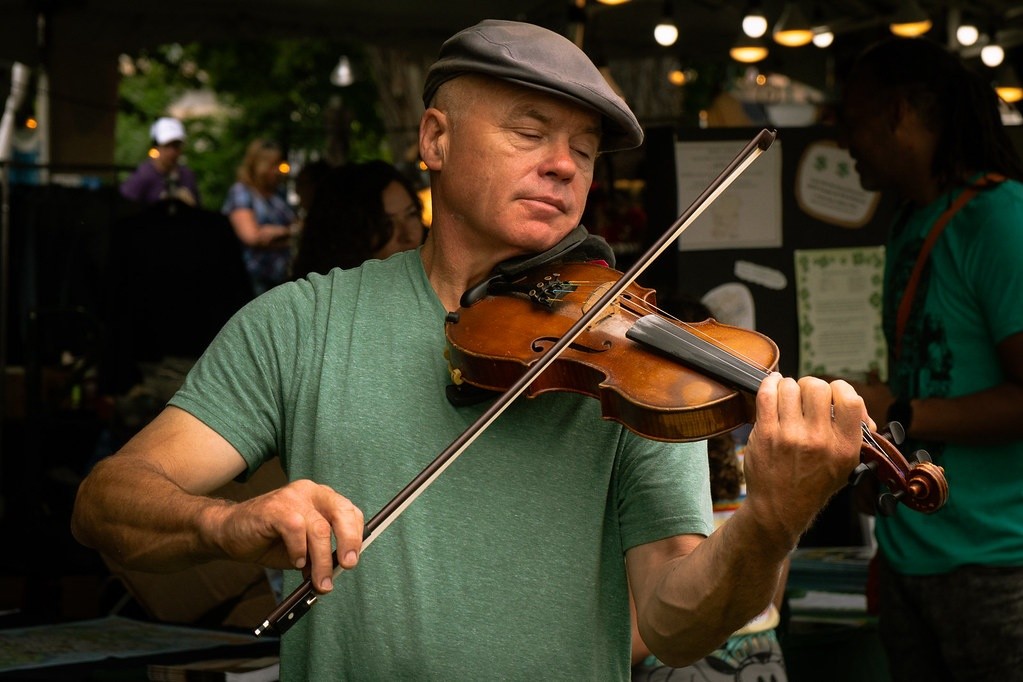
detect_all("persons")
[221,138,306,294]
[811,36,1023,682]
[118,117,205,207]
[293,158,428,282]
[68,16,879,681]
[295,128,349,203]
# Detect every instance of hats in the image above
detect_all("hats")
[422,19,645,152]
[149,116,188,146]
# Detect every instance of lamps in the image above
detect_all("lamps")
[728,0,1023,103]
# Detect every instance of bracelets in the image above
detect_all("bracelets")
[887,393,913,432]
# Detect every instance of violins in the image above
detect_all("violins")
[442,253,949,514]
